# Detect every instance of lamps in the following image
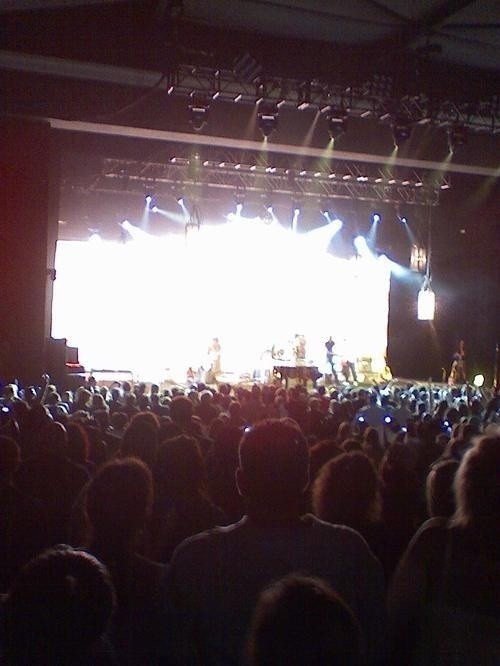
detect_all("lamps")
[183,91,471,151]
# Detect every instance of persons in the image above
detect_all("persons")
[0,366,499,666]
[295,332,307,365]
[207,337,223,376]
[448,337,472,387]
[339,336,360,385]
[324,336,336,379]
